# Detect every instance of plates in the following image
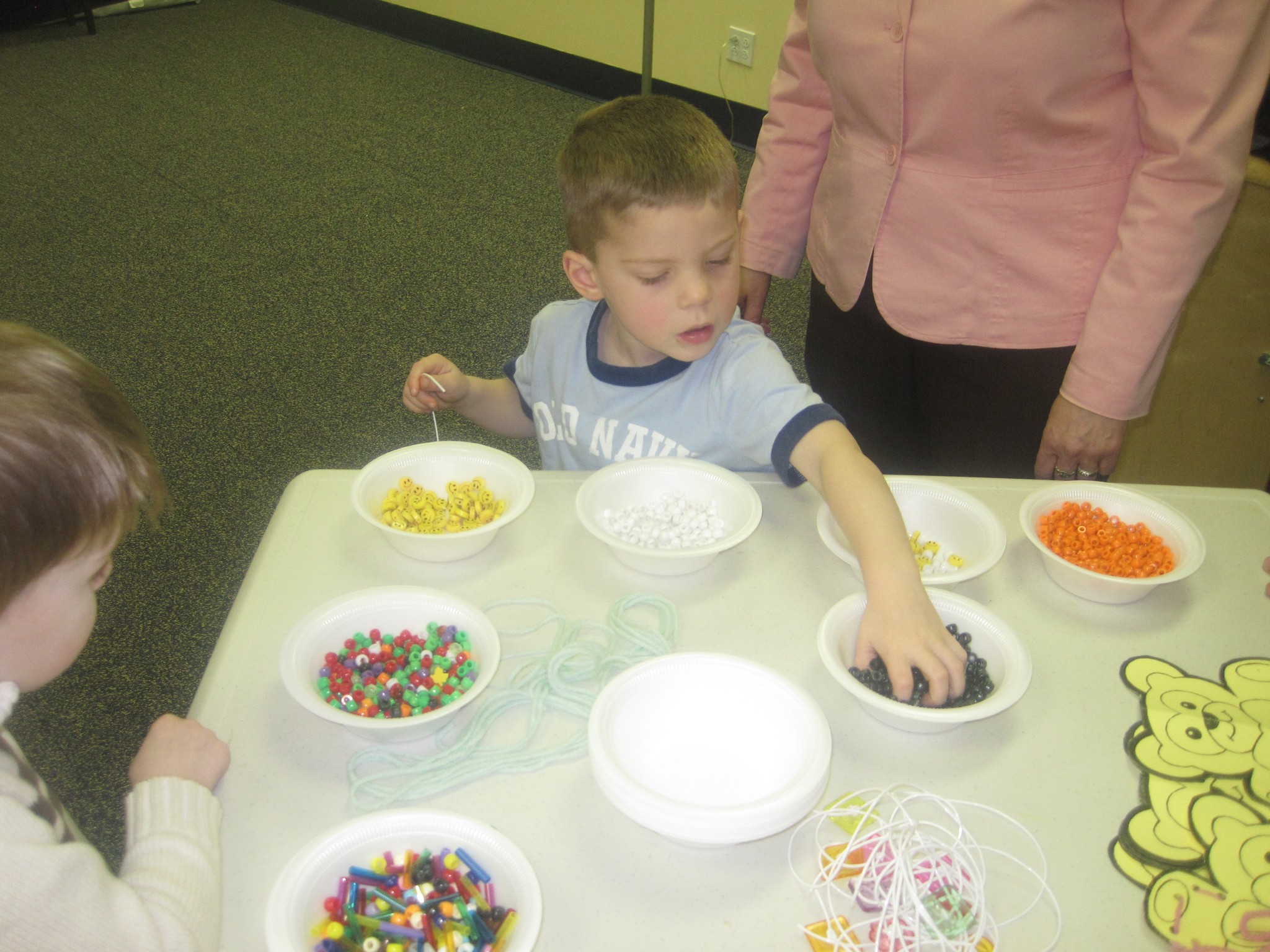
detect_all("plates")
[263,808,543,952]
[585,651,831,849]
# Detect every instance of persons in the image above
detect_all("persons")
[0,305,237,950]
[734,1,1270,488]
[400,93,972,711]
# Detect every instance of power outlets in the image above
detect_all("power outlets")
[726,26,756,67]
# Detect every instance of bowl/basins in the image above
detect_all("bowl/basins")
[268,438,1210,732]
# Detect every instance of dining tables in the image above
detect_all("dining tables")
[182,467,1270,952]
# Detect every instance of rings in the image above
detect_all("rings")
[1053,463,1077,479]
[1077,465,1099,478]
